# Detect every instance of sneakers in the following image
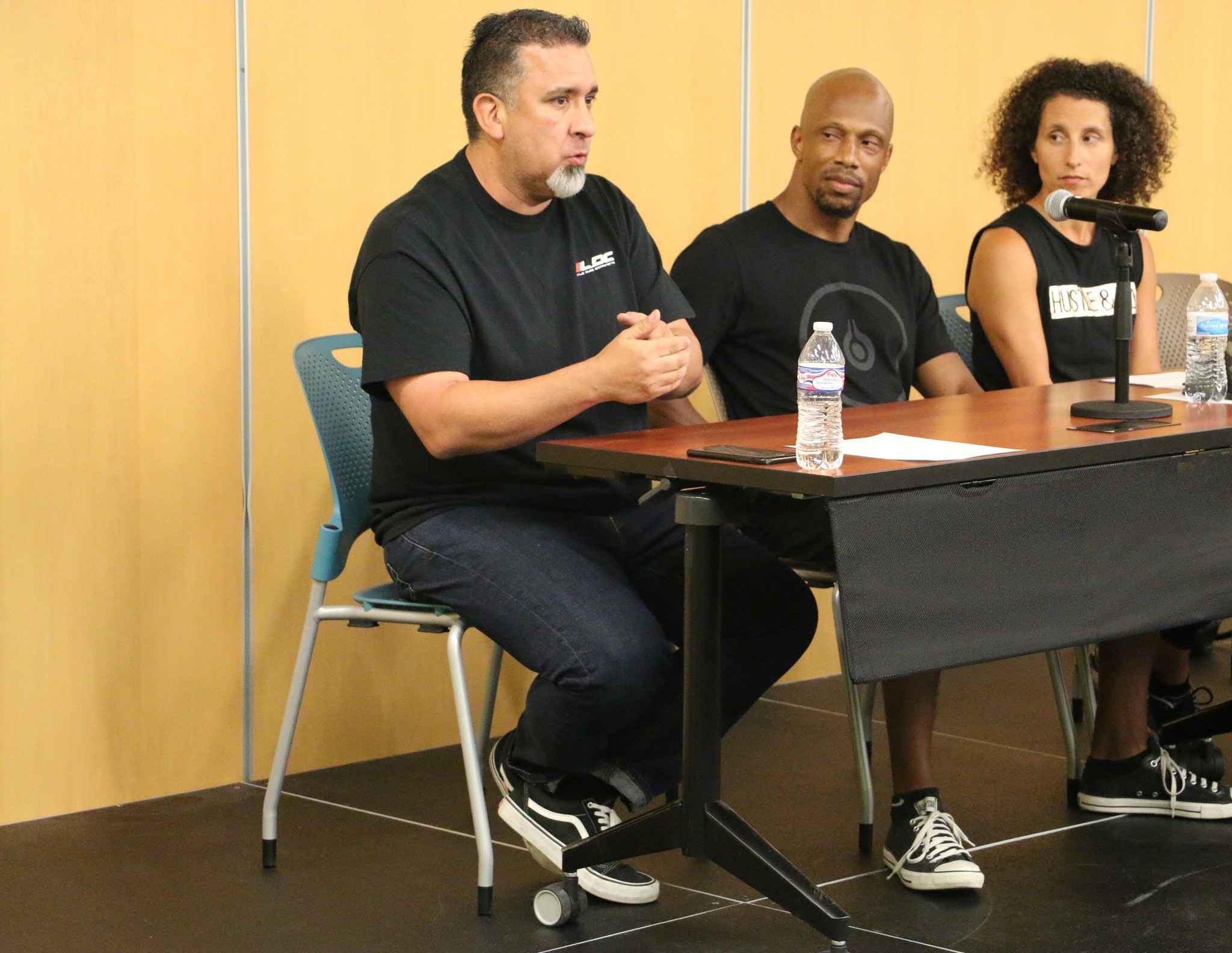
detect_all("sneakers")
[499,781,660,905]
[1146,687,1225,782]
[882,786,985,890]
[488,726,578,877]
[1078,730,1231,820]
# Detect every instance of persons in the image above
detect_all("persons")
[666,58,1232,890]
[348,11,819,907]
[966,61,1225,780]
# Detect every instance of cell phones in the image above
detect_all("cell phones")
[687,444,799,464]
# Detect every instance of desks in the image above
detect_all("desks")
[528,370,1232,953]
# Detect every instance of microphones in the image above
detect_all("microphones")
[1045,189,1168,232]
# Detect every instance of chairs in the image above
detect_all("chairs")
[777,557,1079,854]
[1154,272,1232,369]
[261,333,505,917]
[938,295,1096,747]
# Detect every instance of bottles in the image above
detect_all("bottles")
[1185,274,1230,404]
[796,322,846,469]
